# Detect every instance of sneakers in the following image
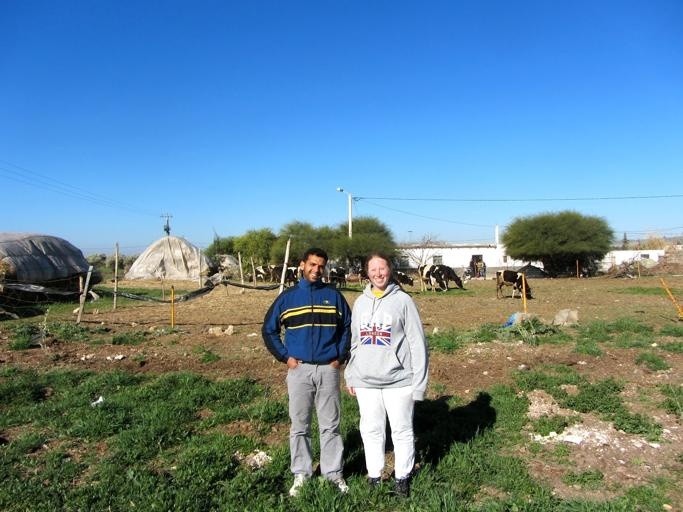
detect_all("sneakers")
[289,475,308,498]
[333,476,350,494]
[395,477,410,497]
[368,477,381,491]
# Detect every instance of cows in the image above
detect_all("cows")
[495,269,533,299]
[394,270,415,287]
[418,263,449,293]
[357,267,369,287]
[436,264,464,290]
[249,264,348,289]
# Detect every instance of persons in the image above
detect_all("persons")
[342,254,428,496]
[257,247,352,496]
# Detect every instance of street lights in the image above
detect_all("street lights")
[337,188,353,240]
